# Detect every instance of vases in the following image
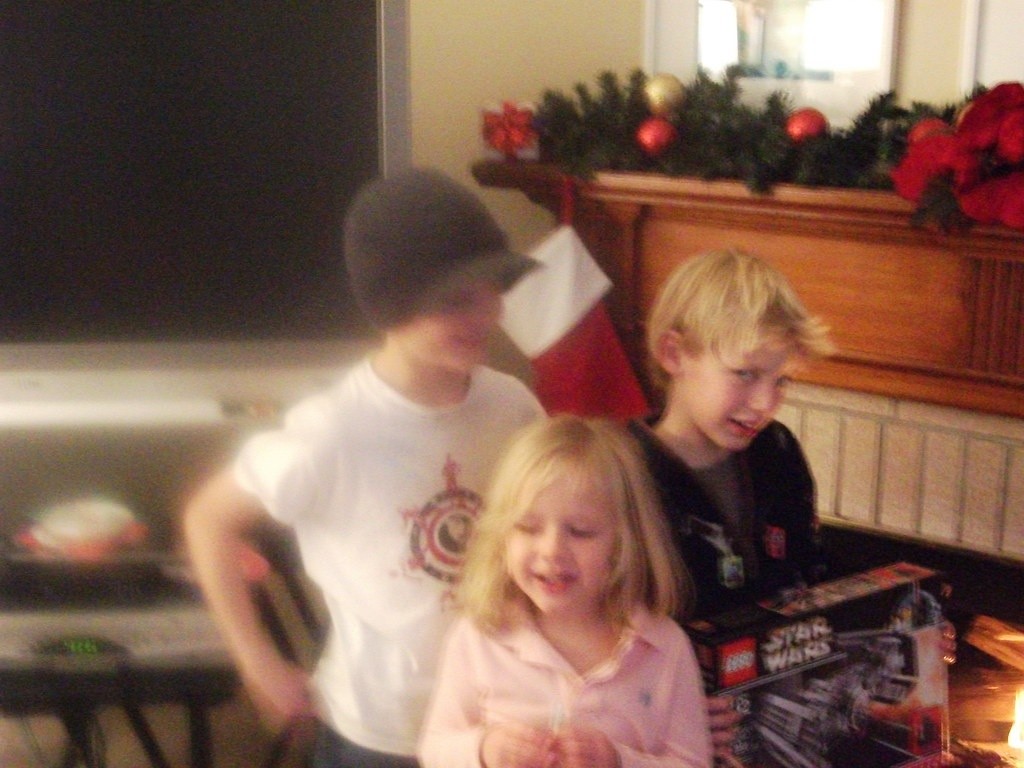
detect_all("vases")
[475,161,1024,420]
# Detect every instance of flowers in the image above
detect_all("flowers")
[481,64,1024,235]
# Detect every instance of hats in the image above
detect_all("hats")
[344,169,542,330]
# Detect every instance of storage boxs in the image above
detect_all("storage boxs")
[681,562,952,768]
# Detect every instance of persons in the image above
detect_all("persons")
[414,414,712,768]
[625,248,957,759]
[184,167,548,768]
